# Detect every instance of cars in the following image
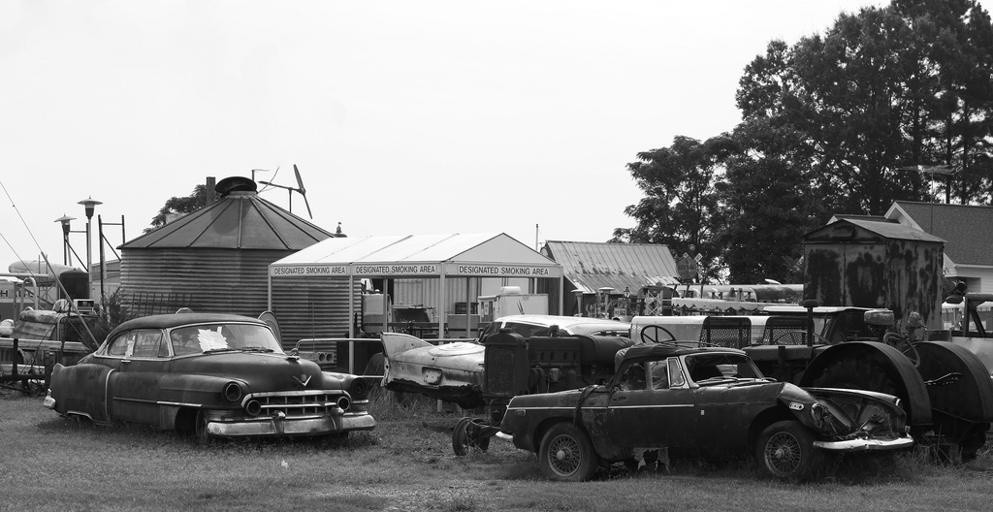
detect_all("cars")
[43,312,377,448]
[494,344,914,483]
[379,312,629,409]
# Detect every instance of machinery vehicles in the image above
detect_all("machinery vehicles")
[451,305,894,458]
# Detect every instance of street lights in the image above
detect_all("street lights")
[52,215,77,265]
[77,196,103,299]
